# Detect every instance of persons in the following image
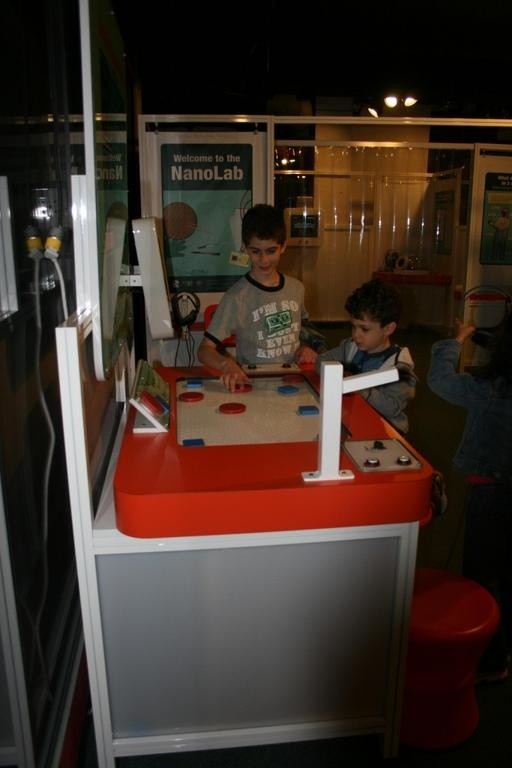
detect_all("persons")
[423,319,510,685]
[313,279,418,448]
[195,203,323,392]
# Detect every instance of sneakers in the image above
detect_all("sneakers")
[430,470,448,517]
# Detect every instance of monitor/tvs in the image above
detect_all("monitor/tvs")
[102,217,127,341]
[131,216,176,340]
[283,208,324,247]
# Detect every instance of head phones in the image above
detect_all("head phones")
[172,291,200,326]
[454,285,512,348]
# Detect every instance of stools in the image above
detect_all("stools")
[402,570,502,749]
